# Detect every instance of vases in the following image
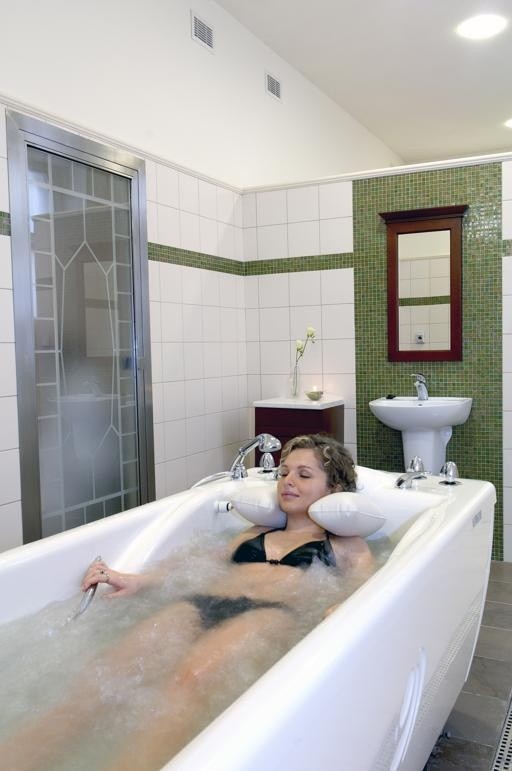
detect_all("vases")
[290,362,304,400]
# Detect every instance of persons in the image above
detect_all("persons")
[0,430,375,770]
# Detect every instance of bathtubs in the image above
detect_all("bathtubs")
[0,464,495,770]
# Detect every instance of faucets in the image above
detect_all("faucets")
[410,373,428,400]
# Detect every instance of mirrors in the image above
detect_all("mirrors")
[386,216,463,362]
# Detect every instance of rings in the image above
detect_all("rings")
[99,569,105,575]
[105,572,111,584]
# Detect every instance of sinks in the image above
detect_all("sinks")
[368,396,473,430]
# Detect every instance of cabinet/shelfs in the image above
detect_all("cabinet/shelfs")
[253,407,343,467]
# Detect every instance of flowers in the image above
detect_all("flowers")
[293,327,316,395]
